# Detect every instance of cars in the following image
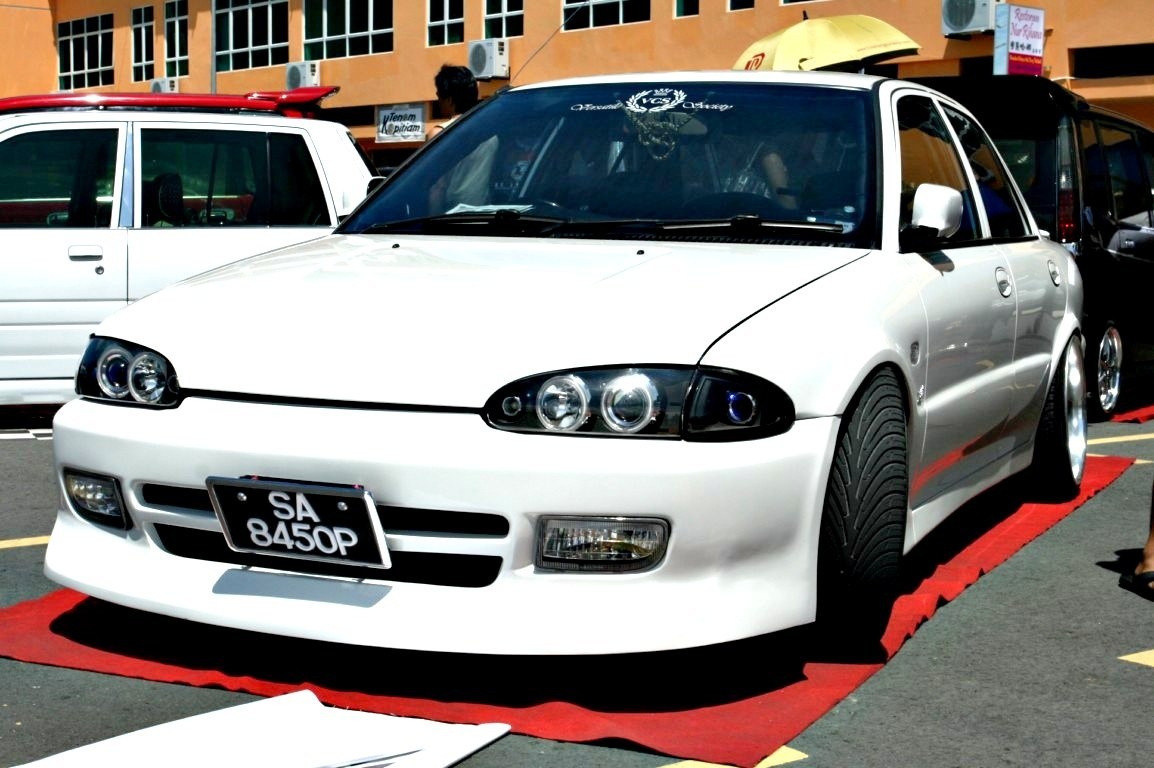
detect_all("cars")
[42,71,1092,657]
[896,73,1154,424]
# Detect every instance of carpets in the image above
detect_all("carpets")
[0,444,1134,768]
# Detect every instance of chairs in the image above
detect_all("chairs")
[146,173,183,226]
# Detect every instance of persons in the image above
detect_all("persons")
[760,89,861,209]
[427,65,498,212]
[148,173,185,228]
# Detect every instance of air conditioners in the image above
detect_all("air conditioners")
[286,61,320,90]
[151,77,179,92]
[942,0,1006,37]
[467,37,510,80]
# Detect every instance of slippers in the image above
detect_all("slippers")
[1119,570,1153,600]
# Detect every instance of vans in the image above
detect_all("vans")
[0,88,381,407]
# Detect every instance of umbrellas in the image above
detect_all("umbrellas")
[731,10,921,74]
